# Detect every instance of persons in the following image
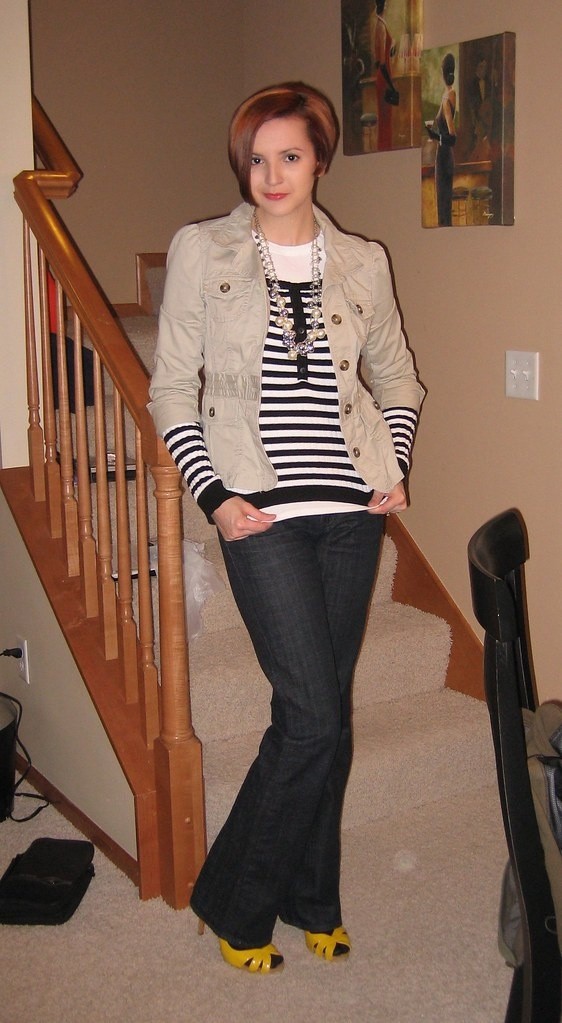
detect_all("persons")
[375,0,400,151]
[431,52,512,227]
[145,82,429,974]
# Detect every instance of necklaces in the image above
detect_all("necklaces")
[254,211,324,356]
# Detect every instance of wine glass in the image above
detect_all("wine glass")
[425,119,435,143]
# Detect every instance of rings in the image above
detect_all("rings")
[386,511,390,517]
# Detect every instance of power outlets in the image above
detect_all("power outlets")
[16,636,29,685]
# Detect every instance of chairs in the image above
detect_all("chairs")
[468,508,562,1023]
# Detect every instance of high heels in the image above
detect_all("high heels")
[305,926,352,962]
[198,917,286,974]
